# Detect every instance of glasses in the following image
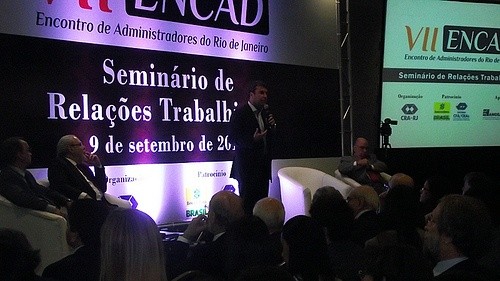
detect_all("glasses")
[424,214,441,226]
[345,198,351,203]
[383,183,392,192]
[71,142,82,146]
[420,187,426,192]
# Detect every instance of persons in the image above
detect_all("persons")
[227,80,277,215]
[0,171,500,281]
[338,137,387,195]
[0,137,74,218]
[48,135,119,212]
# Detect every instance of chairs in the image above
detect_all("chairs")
[334,167,391,187]
[0,191,81,276]
[277,166,352,224]
[37,178,131,209]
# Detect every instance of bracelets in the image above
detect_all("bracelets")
[64,198,71,205]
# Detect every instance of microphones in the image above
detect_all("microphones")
[264,104,273,128]
